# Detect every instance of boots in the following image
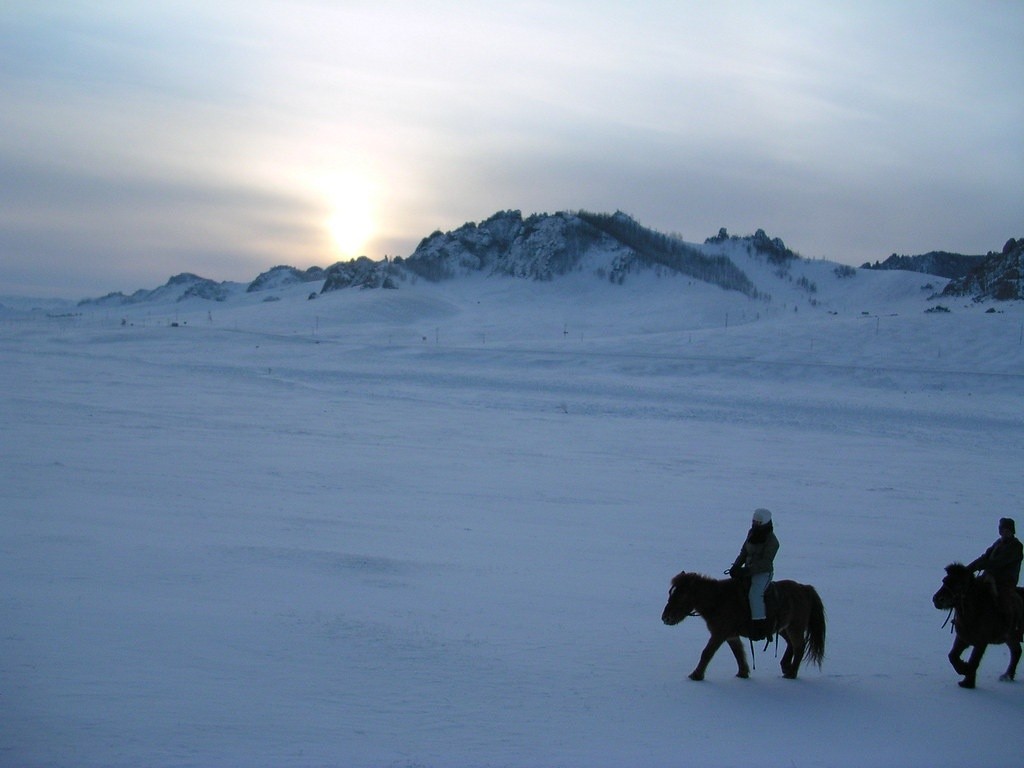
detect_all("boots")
[745,617,769,638]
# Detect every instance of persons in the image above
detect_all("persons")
[967,518,1023,640]
[732,507,780,641]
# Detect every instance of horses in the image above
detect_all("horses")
[660,569,829,681]
[931,562,1024,689]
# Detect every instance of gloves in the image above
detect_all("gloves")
[729,565,744,578]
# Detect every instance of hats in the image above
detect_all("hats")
[999,518,1016,535]
[751,509,772,526]
